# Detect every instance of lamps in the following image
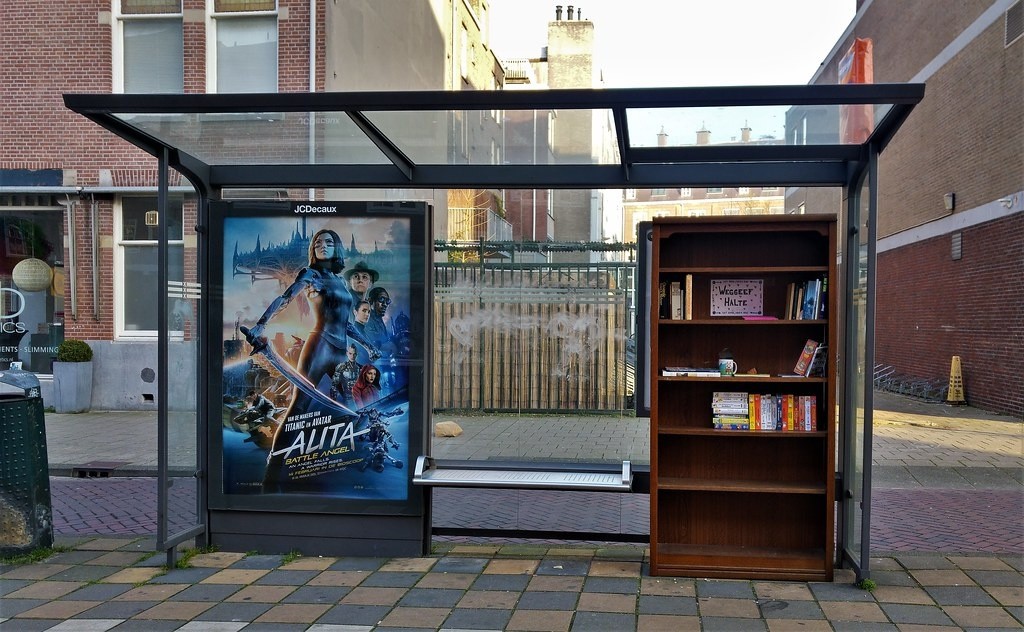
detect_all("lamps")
[12,211,53,292]
[943,192,955,210]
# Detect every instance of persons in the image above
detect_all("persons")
[343,260,392,364]
[251,390,278,425]
[331,343,382,409]
[242,228,382,494]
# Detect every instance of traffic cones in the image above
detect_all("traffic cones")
[945,356,969,405]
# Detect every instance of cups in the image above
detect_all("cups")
[719,359,738,376]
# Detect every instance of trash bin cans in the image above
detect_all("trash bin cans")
[0,367,54,559]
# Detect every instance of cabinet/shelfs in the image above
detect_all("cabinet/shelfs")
[650,213,836,582]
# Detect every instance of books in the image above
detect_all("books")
[660,366,720,376]
[711,392,816,431]
[793,338,829,378]
[734,373,771,377]
[786,274,829,320]
[659,273,693,320]
[742,316,778,321]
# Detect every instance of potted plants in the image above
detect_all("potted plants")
[53,339,94,414]
